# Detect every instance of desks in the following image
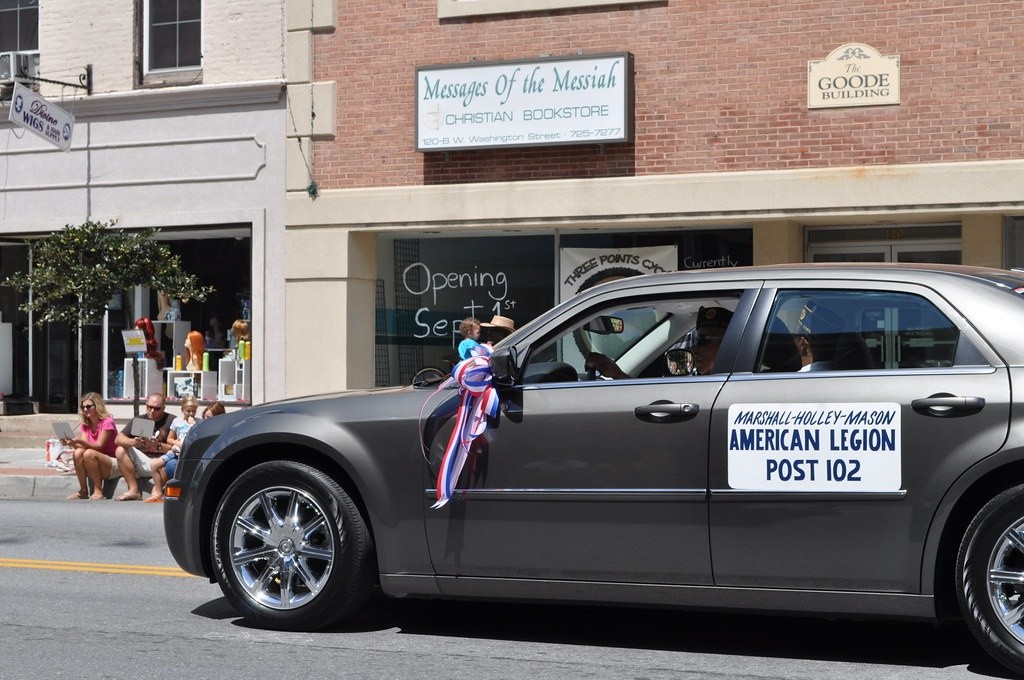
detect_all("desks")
[124,358,164,398]
[167,371,218,400]
[205,349,230,351]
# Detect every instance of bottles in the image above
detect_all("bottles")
[239,337,250,359]
[176,352,181,370]
[202,350,209,371]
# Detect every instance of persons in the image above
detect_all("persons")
[164,401,226,481]
[480,315,517,346]
[184,331,204,371]
[144,396,203,502]
[458,317,480,360]
[791,300,846,372]
[135,317,163,363]
[585,306,734,380]
[230,319,250,348]
[114,393,177,501]
[60,392,131,500]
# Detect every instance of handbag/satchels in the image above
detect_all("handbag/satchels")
[44,438,73,468]
[57,449,77,472]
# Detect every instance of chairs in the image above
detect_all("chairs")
[833,324,875,369]
[763,317,802,373]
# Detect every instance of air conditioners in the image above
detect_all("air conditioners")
[0,52,36,84]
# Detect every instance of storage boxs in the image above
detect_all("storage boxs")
[174,377,199,398]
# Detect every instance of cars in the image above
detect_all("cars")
[161,263,1024,675]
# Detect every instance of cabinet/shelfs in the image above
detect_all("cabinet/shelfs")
[151,321,191,370]
[219,359,252,402]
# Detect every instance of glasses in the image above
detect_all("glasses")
[693,334,723,346]
[147,405,163,413]
[80,404,94,411]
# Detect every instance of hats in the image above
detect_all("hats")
[696,306,734,332]
[479,316,516,333]
[797,300,845,344]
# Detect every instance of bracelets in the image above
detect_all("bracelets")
[612,370,623,379]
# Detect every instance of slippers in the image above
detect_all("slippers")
[114,493,143,500]
[89,494,107,500]
[66,492,90,499]
[143,496,164,503]
